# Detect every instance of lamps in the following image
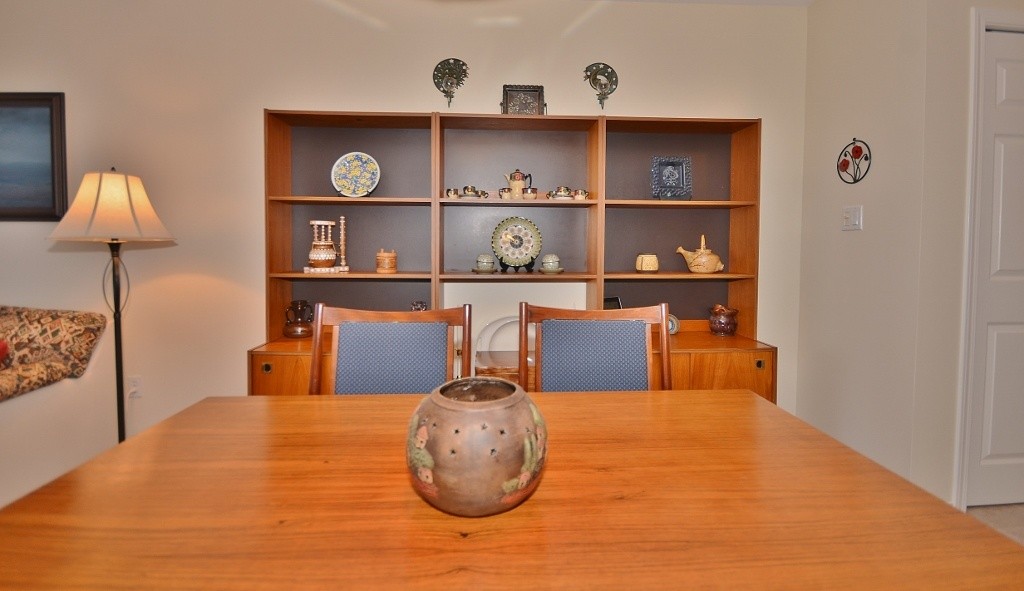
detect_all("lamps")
[47,166,177,443]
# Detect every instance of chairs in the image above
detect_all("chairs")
[309,302,471,395]
[518,302,672,392]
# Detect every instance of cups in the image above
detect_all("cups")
[463,185,475,195]
[546,191,556,200]
[475,190,489,199]
[556,186,571,196]
[476,254,494,270]
[542,254,559,270]
[522,188,538,200]
[376,252,397,273]
[446,188,458,199]
[575,190,589,200]
[635,254,659,274]
[709,306,739,337]
[499,188,512,200]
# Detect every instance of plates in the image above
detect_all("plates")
[472,267,497,275]
[491,216,542,268]
[540,267,565,274]
[330,152,380,197]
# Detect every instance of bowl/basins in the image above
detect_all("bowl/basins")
[406,374,548,518]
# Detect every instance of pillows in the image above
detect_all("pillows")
[0,306,107,379]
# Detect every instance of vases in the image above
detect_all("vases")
[407,376,547,517]
[284,300,315,338]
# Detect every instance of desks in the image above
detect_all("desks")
[1,398,1024,591]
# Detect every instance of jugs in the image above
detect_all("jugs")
[504,169,532,199]
[675,235,724,273]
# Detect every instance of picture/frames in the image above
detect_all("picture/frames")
[503,84,544,115]
[651,155,692,200]
[1,91,68,223]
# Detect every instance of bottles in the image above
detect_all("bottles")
[309,240,337,268]
[284,299,314,338]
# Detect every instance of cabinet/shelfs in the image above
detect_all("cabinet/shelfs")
[247,331,777,406]
[263,108,761,343]
